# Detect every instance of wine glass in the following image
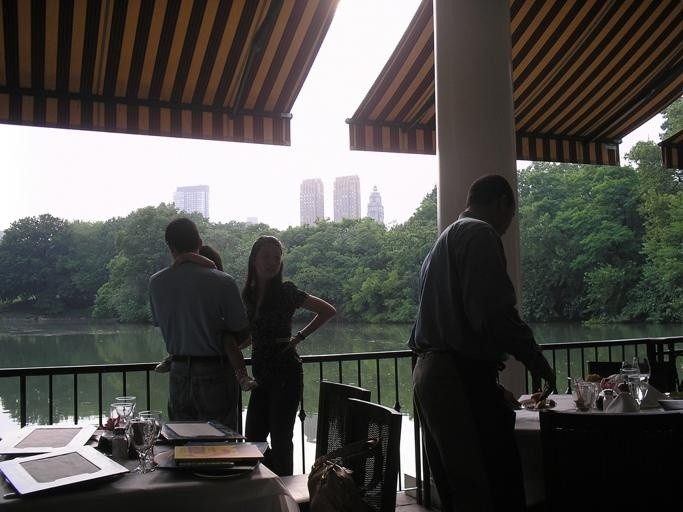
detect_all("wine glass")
[110,395,164,475]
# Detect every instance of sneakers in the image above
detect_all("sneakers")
[237,374,257,392]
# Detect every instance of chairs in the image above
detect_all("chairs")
[623,361,677,394]
[538,408,683,512]
[279,380,371,512]
[587,359,625,382]
[346,396,402,512]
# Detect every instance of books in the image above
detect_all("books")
[156,418,271,473]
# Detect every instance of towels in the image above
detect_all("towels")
[605,391,638,412]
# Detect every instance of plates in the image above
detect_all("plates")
[517,399,557,411]
[189,460,261,479]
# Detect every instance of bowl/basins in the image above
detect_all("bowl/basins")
[657,399,683,412]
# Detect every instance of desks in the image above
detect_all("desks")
[0,419,300,512]
[513,398,683,511]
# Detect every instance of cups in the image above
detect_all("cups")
[570,357,649,412]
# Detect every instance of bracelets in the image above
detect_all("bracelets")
[296,331,305,340]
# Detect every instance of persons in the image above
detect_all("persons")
[153,236,258,392]
[407,174,557,511]
[233,234,337,471]
[147,216,253,441]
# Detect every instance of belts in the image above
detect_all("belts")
[170,352,219,363]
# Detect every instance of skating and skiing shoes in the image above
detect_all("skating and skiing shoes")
[154,356,173,375]
